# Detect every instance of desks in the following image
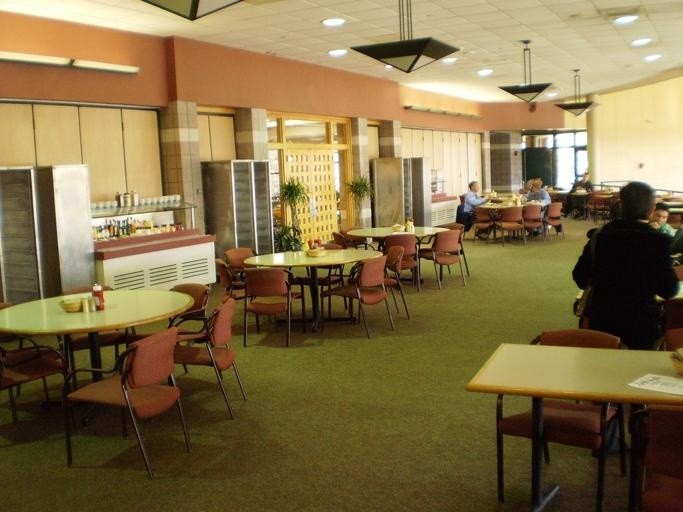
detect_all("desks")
[464,343,683,512]
[2,289,194,420]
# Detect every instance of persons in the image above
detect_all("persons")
[576,181,680,352]
[661,229,682,351]
[647,201,677,240]
[464,181,492,240]
[524,179,551,236]
[571,173,594,219]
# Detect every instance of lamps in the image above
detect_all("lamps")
[141,0,242,22]
[496,39,552,102]
[349,1,462,75]
[553,67,593,118]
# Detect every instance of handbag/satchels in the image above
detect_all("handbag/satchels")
[576,287,593,319]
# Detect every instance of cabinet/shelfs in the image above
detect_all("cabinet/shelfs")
[201,159,274,260]
[0,163,96,306]
[413,157,433,227]
[373,156,413,232]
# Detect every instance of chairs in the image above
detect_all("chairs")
[2,298,62,423]
[629,328,682,511]
[165,297,247,420]
[572,256,682,351]
[459,179,682,244]
[212,218,473,347]
[57,280,137,393]
[64,327,193,480]
[495,329,625,511]
[126,283,210,373]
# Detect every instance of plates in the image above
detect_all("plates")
[576,190,587,194]
[487,190,544,207]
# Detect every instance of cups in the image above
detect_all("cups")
[141,223,184,236]
[140,192,182,205]
[90,200,119,210]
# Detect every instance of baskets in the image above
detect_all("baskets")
[58,297,81,312]
[669,350,683,378]
[392,224,406,232]
[306,248,319,257]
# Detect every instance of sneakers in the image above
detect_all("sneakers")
[592,438,621,458]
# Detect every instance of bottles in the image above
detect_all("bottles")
[91,216,139,241]
[90,284,104,311]
[115,190,139,207]
[306,238,321,251]
[81,297,96,313]
